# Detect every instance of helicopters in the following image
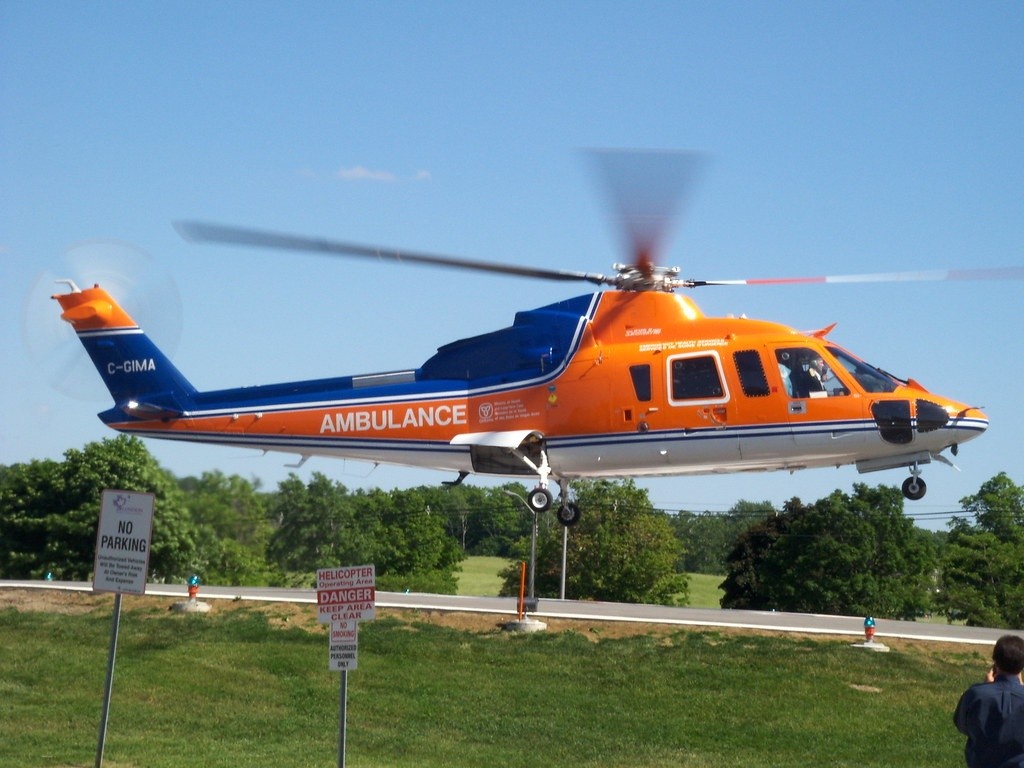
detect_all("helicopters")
[50,145,1023,528]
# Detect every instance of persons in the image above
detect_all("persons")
[953,635,1024,768]
[800,351,828,398]
